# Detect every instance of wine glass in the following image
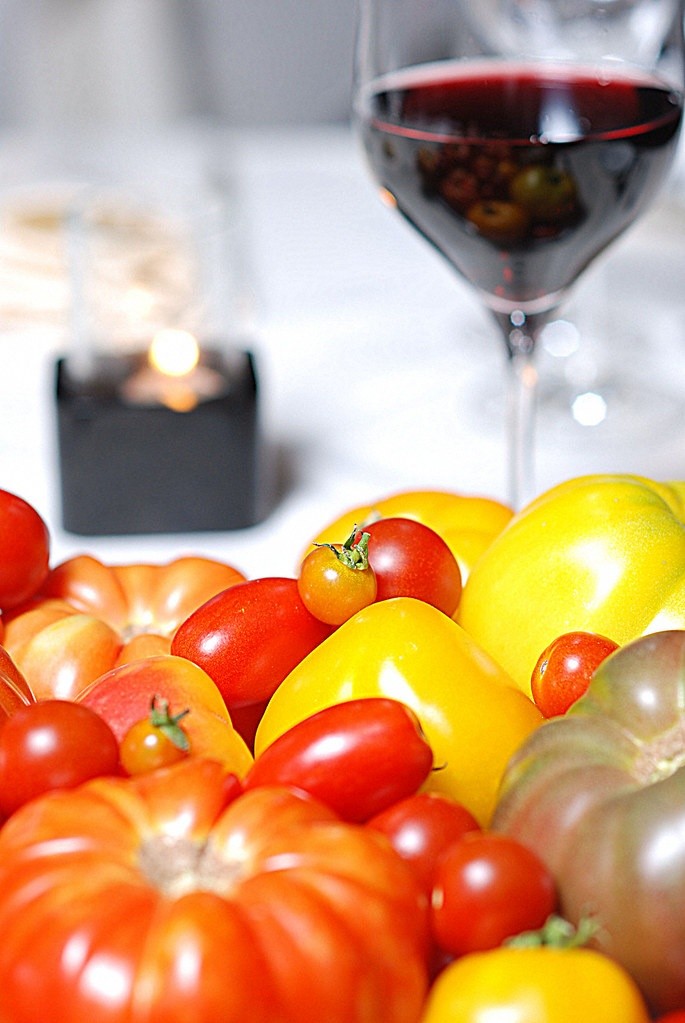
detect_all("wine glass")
[350,1,684,525]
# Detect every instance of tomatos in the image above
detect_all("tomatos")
[0,473,685,1023]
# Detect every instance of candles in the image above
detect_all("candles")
[56,333,261,537]
